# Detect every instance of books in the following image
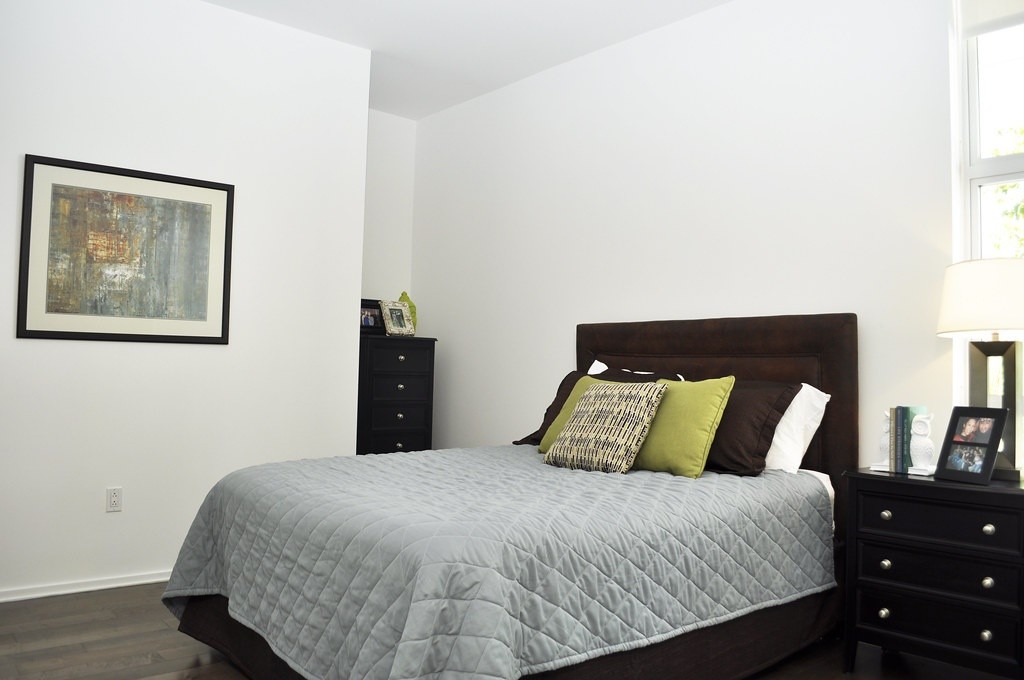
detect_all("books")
[889,406,912,473]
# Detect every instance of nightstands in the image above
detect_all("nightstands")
[843,467,1024,680]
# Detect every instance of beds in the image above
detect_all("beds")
[160,312,858,680]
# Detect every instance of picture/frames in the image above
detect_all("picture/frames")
[16,153,235,346]
[380,300,415,336]
[934,406,1009,485]
[361,298,386,335]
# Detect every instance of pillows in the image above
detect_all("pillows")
[508,360,832,477]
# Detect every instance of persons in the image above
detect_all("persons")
[948,444,985,473]
[361,311,374,325]
[952,416,994,443]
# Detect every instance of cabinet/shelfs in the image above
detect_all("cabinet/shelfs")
[356,335,438,455]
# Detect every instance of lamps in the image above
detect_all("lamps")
[936,256,1024,481]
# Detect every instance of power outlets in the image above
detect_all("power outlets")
[106,487,123,512]
